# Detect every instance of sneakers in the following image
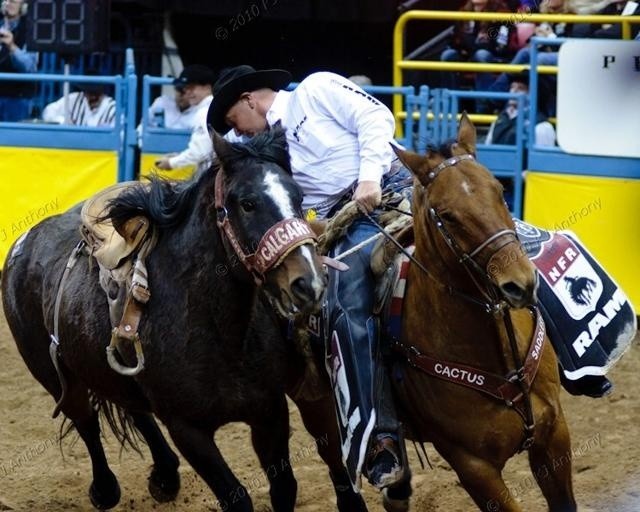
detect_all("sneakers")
[559,363,611,397]
[365,437,400,485]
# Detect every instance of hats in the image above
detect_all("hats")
[207,64,292,137]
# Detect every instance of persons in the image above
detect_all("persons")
[155,63,244,178]
[0,1,42,120]
[484,68,559,209]
[438,1,639,111]
[207,62,612,487]
[136,74,193,148]
[42,71,116,127]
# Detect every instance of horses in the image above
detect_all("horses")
[0,118,330,512]
[288,110,577,512]
